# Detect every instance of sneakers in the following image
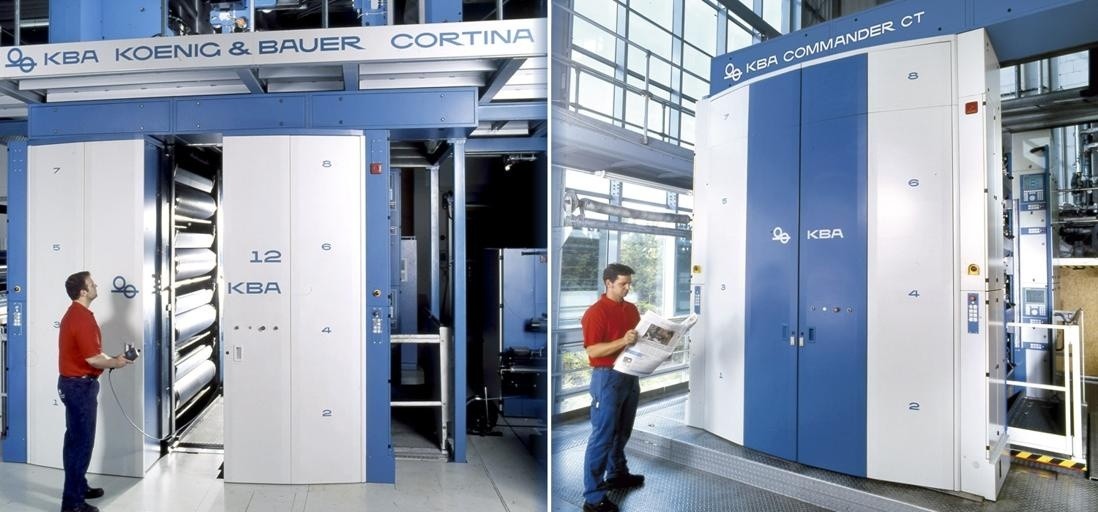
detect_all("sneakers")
[606,473,644,488]
[83,486,104,498]
[583,496,620,512]
[61,503,99,512]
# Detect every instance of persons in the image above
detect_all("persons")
[579,262,648,512]
[56,271,138,512]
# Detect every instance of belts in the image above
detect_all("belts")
[60,373,99,380]
[594,365,612,372]
